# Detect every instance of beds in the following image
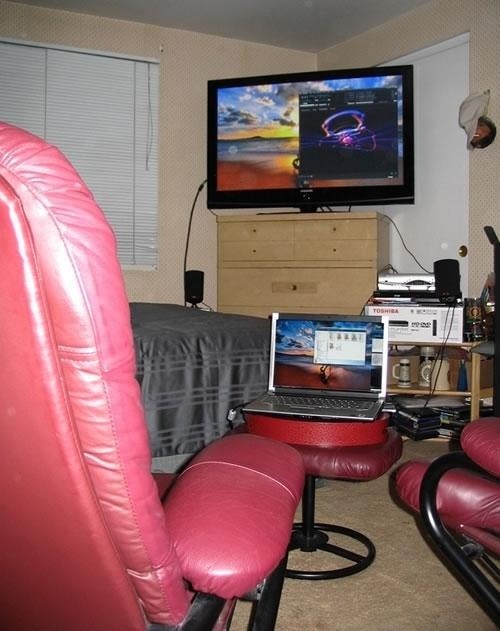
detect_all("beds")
[126,301,272,475]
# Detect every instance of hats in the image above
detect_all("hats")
[458,90,490,151]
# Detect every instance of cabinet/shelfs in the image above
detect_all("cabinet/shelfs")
[215,212,391,320]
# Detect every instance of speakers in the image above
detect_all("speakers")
[434,259,462,303]
[184,270,206,308]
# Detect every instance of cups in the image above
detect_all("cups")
[420,359,452,392]
[392,358,412,389]
[417,345,436,387]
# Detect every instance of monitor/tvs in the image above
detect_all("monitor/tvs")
[203,63,415,214]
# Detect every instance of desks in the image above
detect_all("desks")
[385,340,497,425]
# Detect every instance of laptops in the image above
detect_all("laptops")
[241,311,390,423]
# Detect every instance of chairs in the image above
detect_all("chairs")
[0,116,303,631]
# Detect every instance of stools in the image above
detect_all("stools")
[226,419,406,582]
